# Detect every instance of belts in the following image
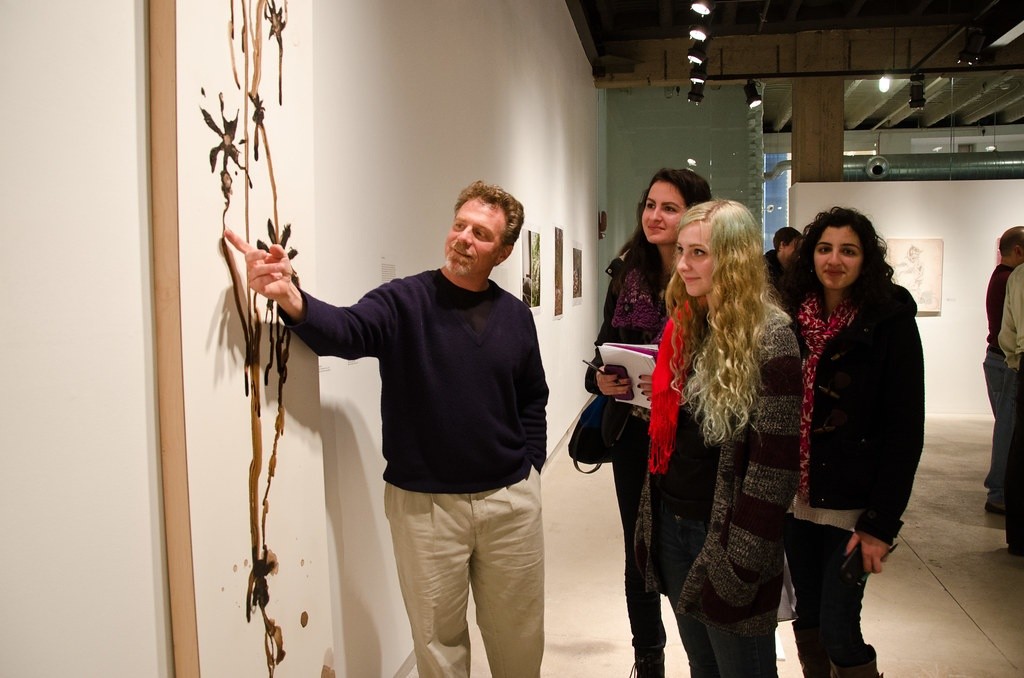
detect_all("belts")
[988,346,1006,357]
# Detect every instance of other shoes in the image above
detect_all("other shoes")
[985,501,1007,515]
[1008,543,1024,555]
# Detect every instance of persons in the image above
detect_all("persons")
[780,207,925,678]
[998,263,1024,556]
[763,227,803,289]
[983,226,1024,515]
[222,181,550,678]
[634,200,805,678]
[585,168,720,678]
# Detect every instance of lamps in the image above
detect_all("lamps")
[687,36,710,65]
[908,74,926,108]
[688,0,719,17]
[688,12,718,42]
[687,78,706,103]
[743,79,763,109]
[664,85,673,98]
[689,56,707,84]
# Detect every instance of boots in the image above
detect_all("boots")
[795,625,830,678]
[629,653,664,678]
[831,644,884,677]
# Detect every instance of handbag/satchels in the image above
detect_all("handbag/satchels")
[568,395,636,473]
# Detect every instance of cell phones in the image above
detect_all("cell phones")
[604,364,634,401]
[841,544,867,586]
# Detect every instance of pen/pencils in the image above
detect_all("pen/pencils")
[582,359,622,387]
